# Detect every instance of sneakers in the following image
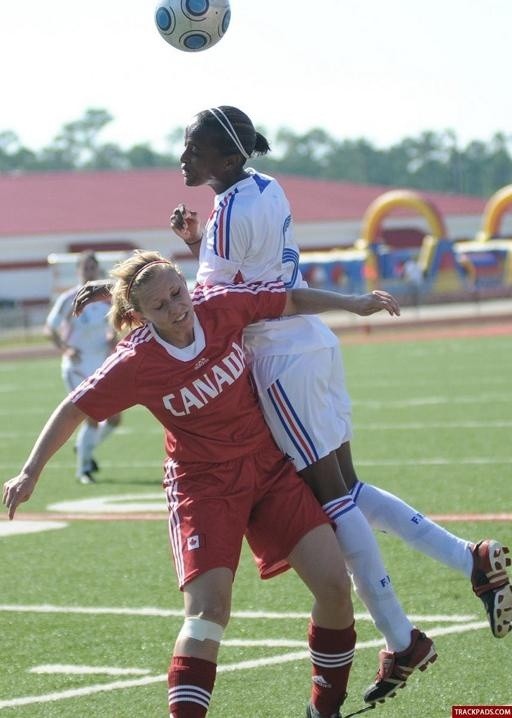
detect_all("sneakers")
[468,539,512,638]
[72,446,99,484]
[364,629,438,706]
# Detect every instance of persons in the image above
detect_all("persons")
[3,249,400,718]
[71,103,510,705]
[42,253,122,486]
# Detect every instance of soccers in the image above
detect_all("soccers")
[155,0,231,52]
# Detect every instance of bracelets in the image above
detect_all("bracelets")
[184,231,205,246]
